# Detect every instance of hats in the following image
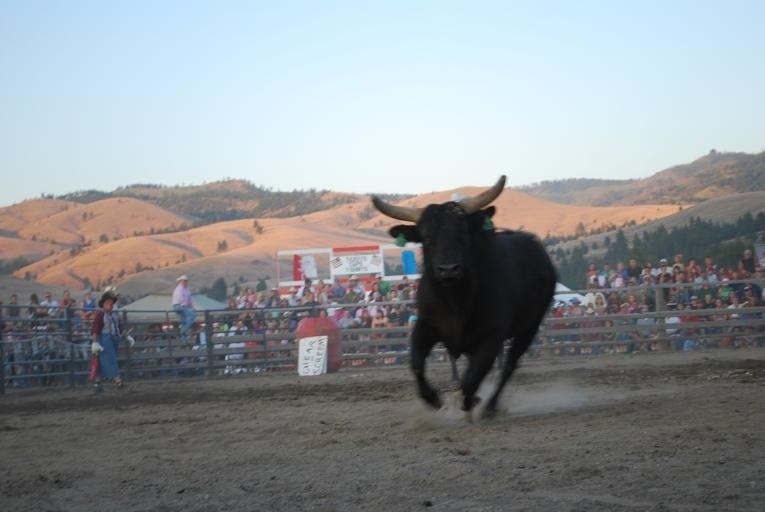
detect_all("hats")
[177,274,188,282]
[83,289,91,296]
[228,274,421,326]
[40,301,49,307]
[30,294,38,299]
[10,294,17,300]
[44,292,51,297]
[98,293,117,307]
[552,251,765,326]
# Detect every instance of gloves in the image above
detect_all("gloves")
[126,336,136,349]
[91,342,105,357]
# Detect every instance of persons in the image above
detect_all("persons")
[4,289,126,391]
[525,250,765,354]
[128,276,420,377]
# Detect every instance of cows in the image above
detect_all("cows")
[369,174,558,419]
